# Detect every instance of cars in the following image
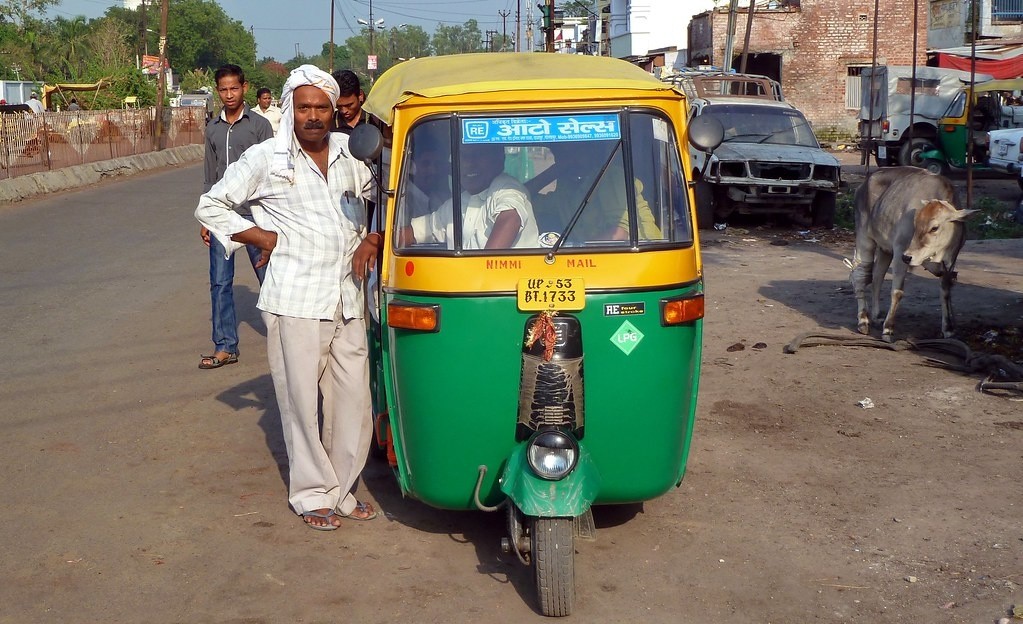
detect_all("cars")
[0,75,214,156]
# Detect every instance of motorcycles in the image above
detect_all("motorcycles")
[346,52,726,618]
[854,63,1023,190]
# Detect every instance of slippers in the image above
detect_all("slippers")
[347,500,376,519]
[303,508,339,530]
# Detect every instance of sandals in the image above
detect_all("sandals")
[198,352,238,368]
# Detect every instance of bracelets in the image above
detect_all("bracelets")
[368,232,382,244]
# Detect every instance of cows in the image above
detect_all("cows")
[848,166,981,344]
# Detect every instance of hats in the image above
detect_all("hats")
[31,92,38,97]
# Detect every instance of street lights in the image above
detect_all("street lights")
[357,0,385,90]
[376,23,407,67]
[146,28,168,152]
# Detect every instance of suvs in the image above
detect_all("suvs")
[683,74,841,230]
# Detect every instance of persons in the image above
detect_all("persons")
[199,64,268,369]
[524,142,662,241]
[250,70,449,233]
[194,64,378,530]
[352,141,541,282]
